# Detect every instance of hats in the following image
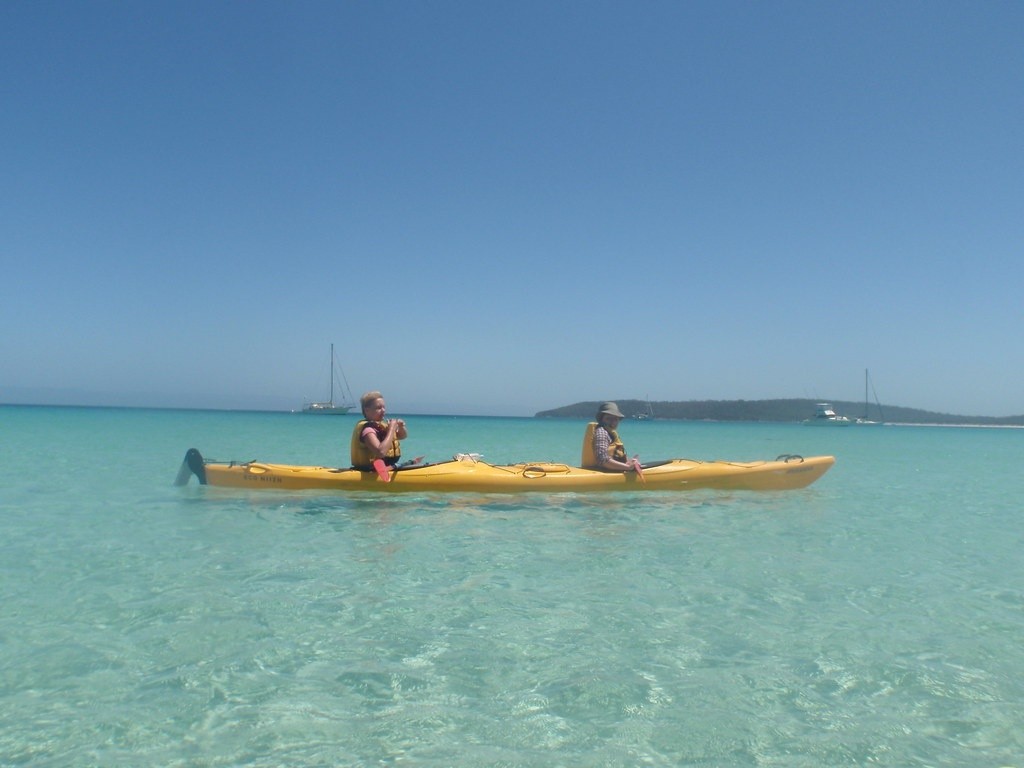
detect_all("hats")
[596,402,625,424]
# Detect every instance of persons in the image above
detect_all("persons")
[582,402,641,470]
[351,392,408,471]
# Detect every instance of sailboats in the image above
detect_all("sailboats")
[303,343,357,415]
[852,368,884,425]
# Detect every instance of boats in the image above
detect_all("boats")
[186,448,835,493]
[803,403,851,426]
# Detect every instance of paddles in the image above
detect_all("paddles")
[373,455,423,482]
[631,454,646,485]
